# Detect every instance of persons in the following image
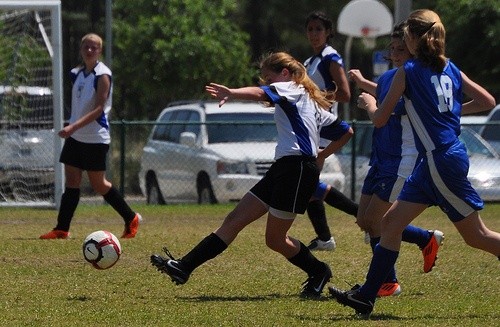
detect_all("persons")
[149,52,354,302]
[300,9,372,251]
[348,22,445,298]
[41,31,142,239]
[326,8,500,318]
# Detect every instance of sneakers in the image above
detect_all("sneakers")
[421,230,445,273]
[328,283,373,319]
[377,281,400,297]
[151,247,190,286]
[300,262,334,300]
[39,230,69,239]
[306,236,335,250]
[121,212,142,238]
[364,230,370,244]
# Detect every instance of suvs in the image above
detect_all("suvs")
[138,99,347,205]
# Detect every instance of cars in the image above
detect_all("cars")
[334,104,500,211]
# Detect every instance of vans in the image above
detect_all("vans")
[0,85,73,171]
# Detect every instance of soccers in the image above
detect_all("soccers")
[83,230,121,270]
